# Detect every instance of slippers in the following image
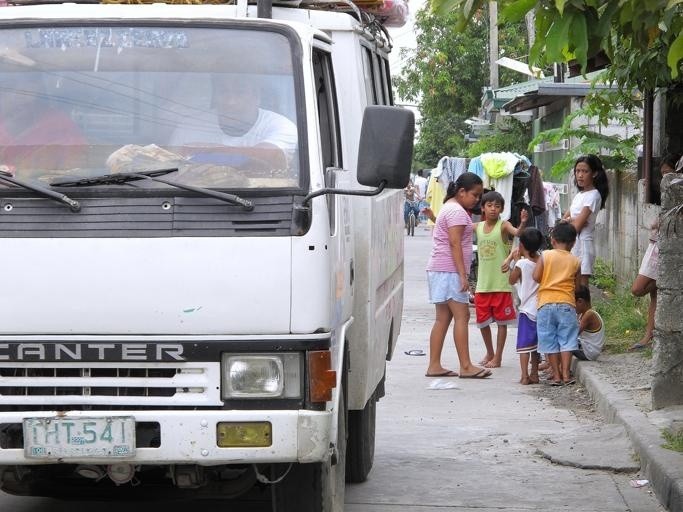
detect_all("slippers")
[425,367,494,378]
[628,341,649,352]
[549,377,575,386]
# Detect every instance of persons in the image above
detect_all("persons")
[403,183,422,229]
[421,192,529,369]
[422,170,495,380]
[532,220,583,387]
[631,151,683,350]
[559,152,610,296]
[167,51,298,176]
[0,64,90,186]
[506,227,545,386]
[535,283,606,374]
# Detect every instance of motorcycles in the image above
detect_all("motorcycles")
[405,198,422,235]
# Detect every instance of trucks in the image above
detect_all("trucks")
[0,5,415,511]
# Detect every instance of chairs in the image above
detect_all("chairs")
[211,86,281,114]
